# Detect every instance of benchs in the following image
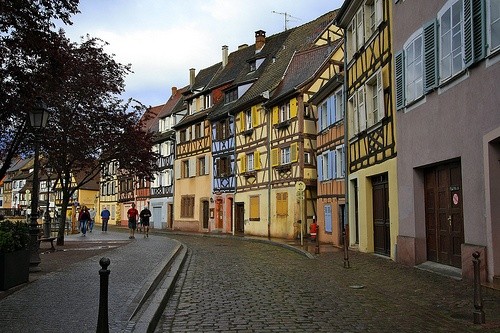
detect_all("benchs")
[35,237,56,253]
[38,222,69,236]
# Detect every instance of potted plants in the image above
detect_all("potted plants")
[0,220,31,292]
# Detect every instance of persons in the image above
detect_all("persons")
[37,207,60,222]
[78,205,96,237]
[127,203,139,239]
[139,205,151,236]
[101,207,110,231]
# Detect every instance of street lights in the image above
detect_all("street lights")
[41,158,52,240]
[25,94,52,271]
[14,195,17,214]
